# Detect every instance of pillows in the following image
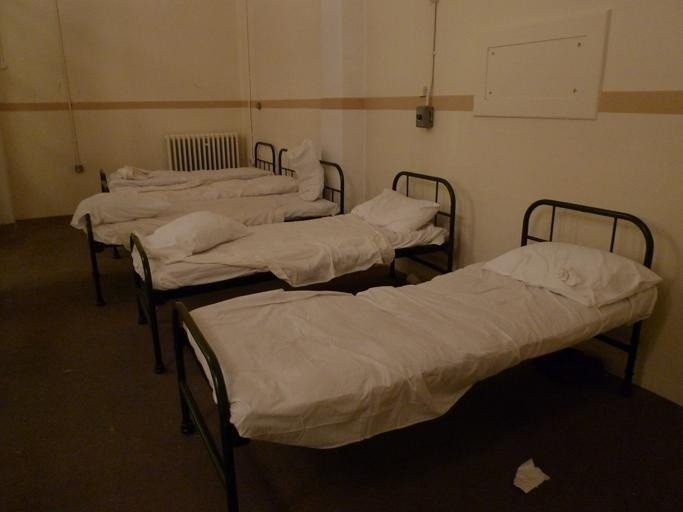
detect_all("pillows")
[352,186,445,237]
[480,239,662,309]
[278,143,331,201]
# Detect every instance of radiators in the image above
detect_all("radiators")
[163,131,238,173]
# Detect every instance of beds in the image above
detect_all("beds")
[180,196,654,510]
[84,147,351,294]
[92,143,281,193]
[120,171,463,376]
